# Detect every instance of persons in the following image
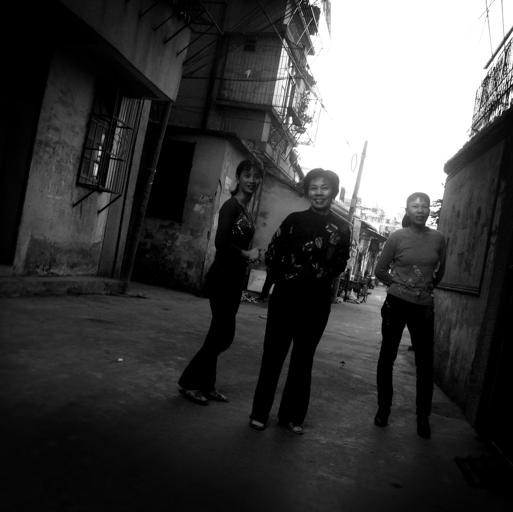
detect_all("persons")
[249,168,353,438]
[375,192,448,437]
[176,159,270,407]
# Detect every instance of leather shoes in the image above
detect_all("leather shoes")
[374,408,390,427]
[176,383,209,406]
[416,414,430,439]
[206,390,228,402]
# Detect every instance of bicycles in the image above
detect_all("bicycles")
[356,280,371,303]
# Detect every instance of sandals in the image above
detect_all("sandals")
[249,418,265,430]
[286,422,304,435]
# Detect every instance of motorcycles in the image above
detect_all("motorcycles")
[367,275,376,289]
